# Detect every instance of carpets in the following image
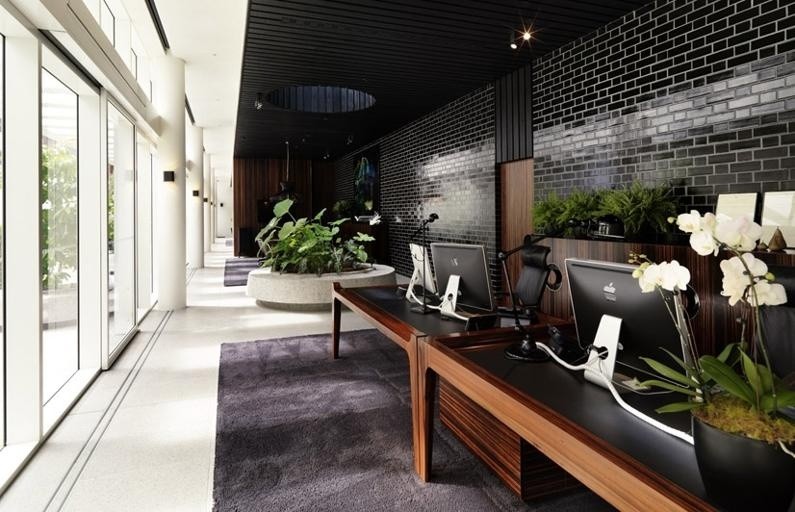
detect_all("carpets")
[223,254,264,287]
[206,329,608,511]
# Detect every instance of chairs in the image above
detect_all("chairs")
[512,246,551,309]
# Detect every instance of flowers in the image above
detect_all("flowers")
[624,210,793,451]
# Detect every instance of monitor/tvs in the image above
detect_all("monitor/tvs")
[406,242,437,304]
[564,257,695,395]
[429,242,495,321]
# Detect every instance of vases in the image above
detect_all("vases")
[691,404,793,509]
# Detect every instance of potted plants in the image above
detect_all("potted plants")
[245,197,398,311]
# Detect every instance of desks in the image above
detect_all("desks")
[325,282,533,483]
[411,329,691,512]
